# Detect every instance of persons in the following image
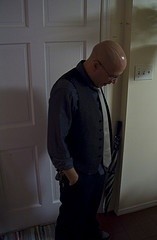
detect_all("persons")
[47,41,127,240]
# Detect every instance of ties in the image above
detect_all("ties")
[98,88,111,168]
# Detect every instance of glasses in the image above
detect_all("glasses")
[95,60,118,78]
